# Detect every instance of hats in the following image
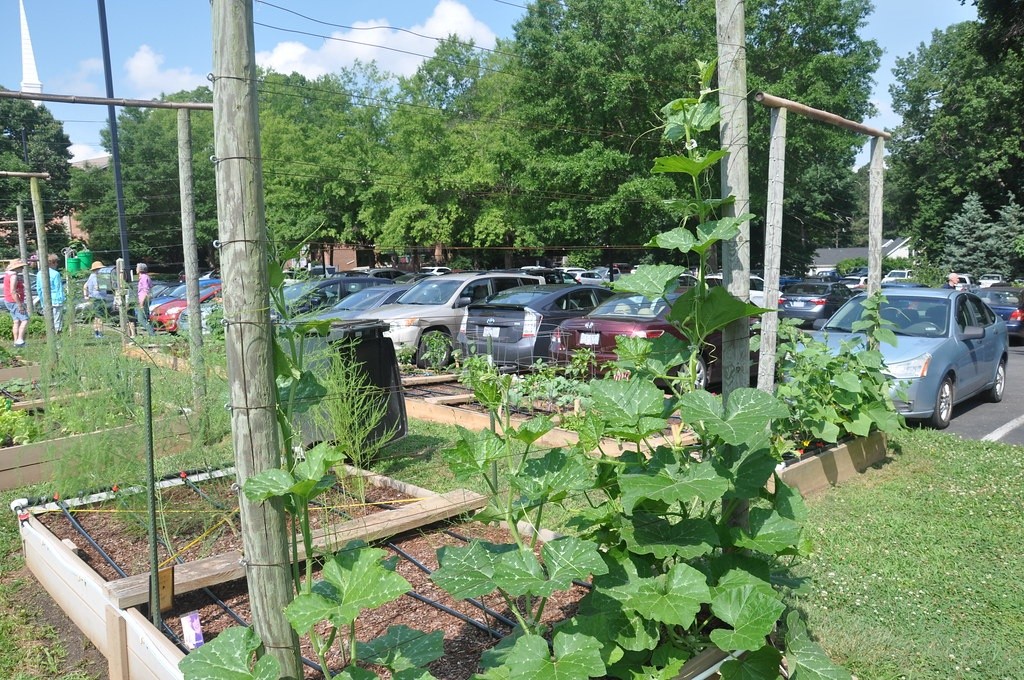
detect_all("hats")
[5,258,27,270]
[90,261,106,271]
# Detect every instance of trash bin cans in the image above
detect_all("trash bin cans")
[297,318,407,463]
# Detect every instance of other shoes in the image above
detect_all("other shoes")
[14,341,26,347]
[96,334,107,339]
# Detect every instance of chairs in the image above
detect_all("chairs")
[613,303,631,315]
[636,308,654,316]
[897,308,920,327]
[925,306,947,333]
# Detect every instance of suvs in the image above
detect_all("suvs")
[334,265,549,372]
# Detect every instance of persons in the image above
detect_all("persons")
[136,263,154,335]
[128,287,138,338]
[84,261,106,338]
[942,273,959,290]
[4,258,29,347]
[36,254,64,334]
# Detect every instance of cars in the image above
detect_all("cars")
[72,260,765,343]
[780,286,1009,430]
[454,284,641,374]
[777,264,1024,344]
[547,285,785,396]
[0,272,68,316]
